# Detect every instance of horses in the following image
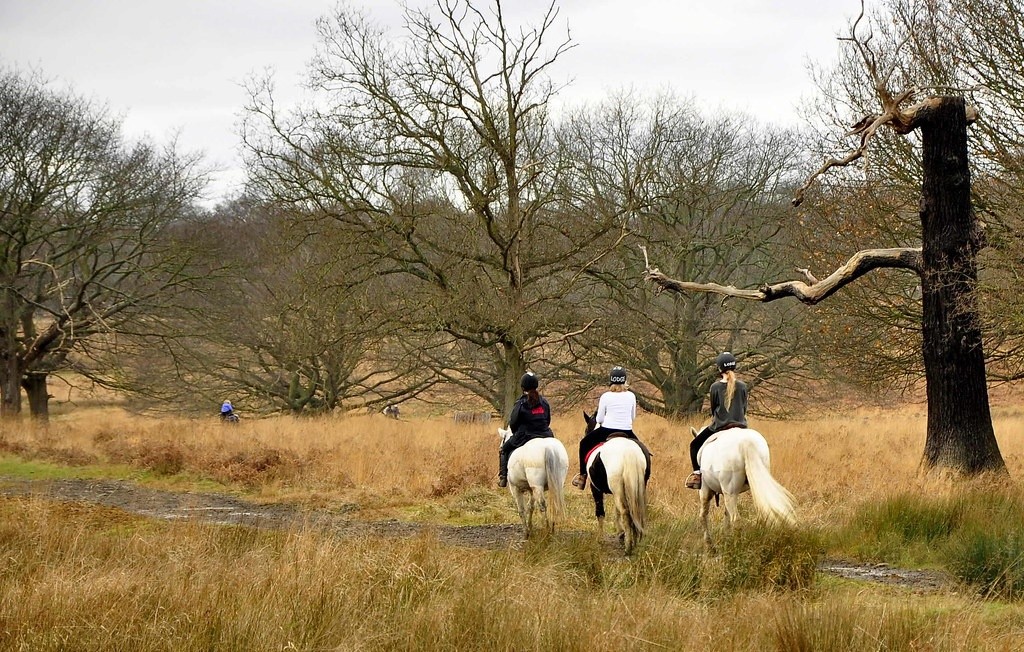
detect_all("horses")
[579,409,647,557]
[497,422,569,546]
[688,424,803,553]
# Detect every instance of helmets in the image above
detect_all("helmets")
[717,352,737,370]
[610,366,627,384]
[520,372,539,389]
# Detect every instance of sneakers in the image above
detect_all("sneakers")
[498,476,507,487]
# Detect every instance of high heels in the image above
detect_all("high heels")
[685,473,702,489]
[572,473,587,490]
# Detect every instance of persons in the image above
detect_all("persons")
[497,373,554,487]
[686,352,747,489]
[383,405,400,419]
[221,400,233,416]
[572,366,636,490]
